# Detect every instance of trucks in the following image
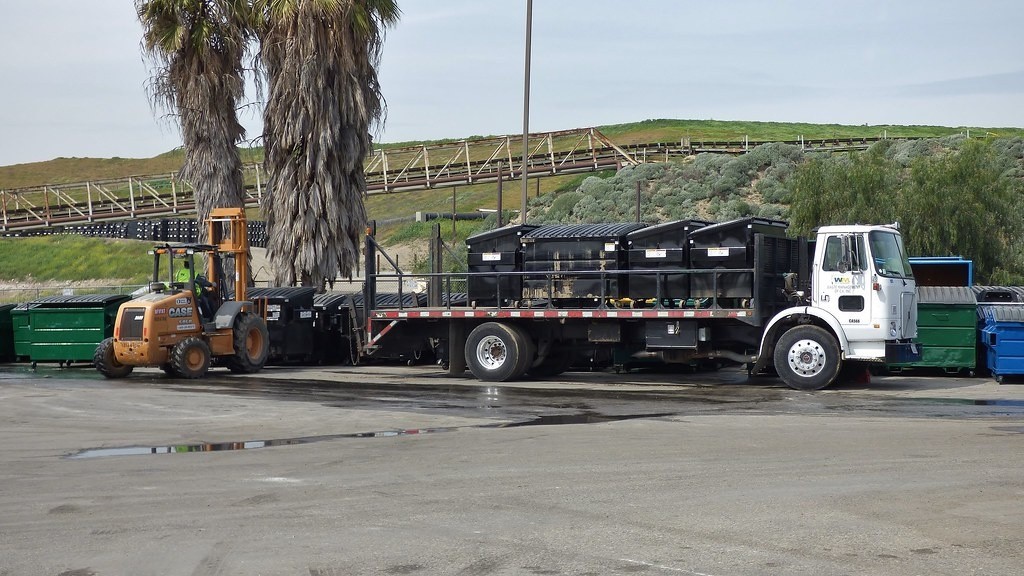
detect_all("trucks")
[349,181,923,393]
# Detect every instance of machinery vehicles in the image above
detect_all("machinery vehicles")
[94,206,272,378]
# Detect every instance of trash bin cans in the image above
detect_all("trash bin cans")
[224,288,467,364]
[0,292,132,370]
[882,281,1024,386]
[465,214,790,310]
